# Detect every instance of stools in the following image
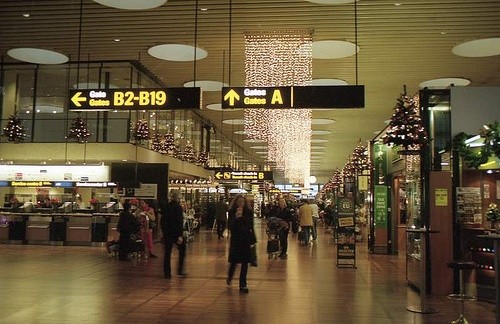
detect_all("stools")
[447,261,479,324]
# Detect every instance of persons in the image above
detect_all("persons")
[160,189,189,279]
[205,196,228,239]
[267,217,289,257]
[117,203,138,262]
[261,198,333,246]
[42,198,52,208]
[226,195,258,294]
[140,199,157,258]
